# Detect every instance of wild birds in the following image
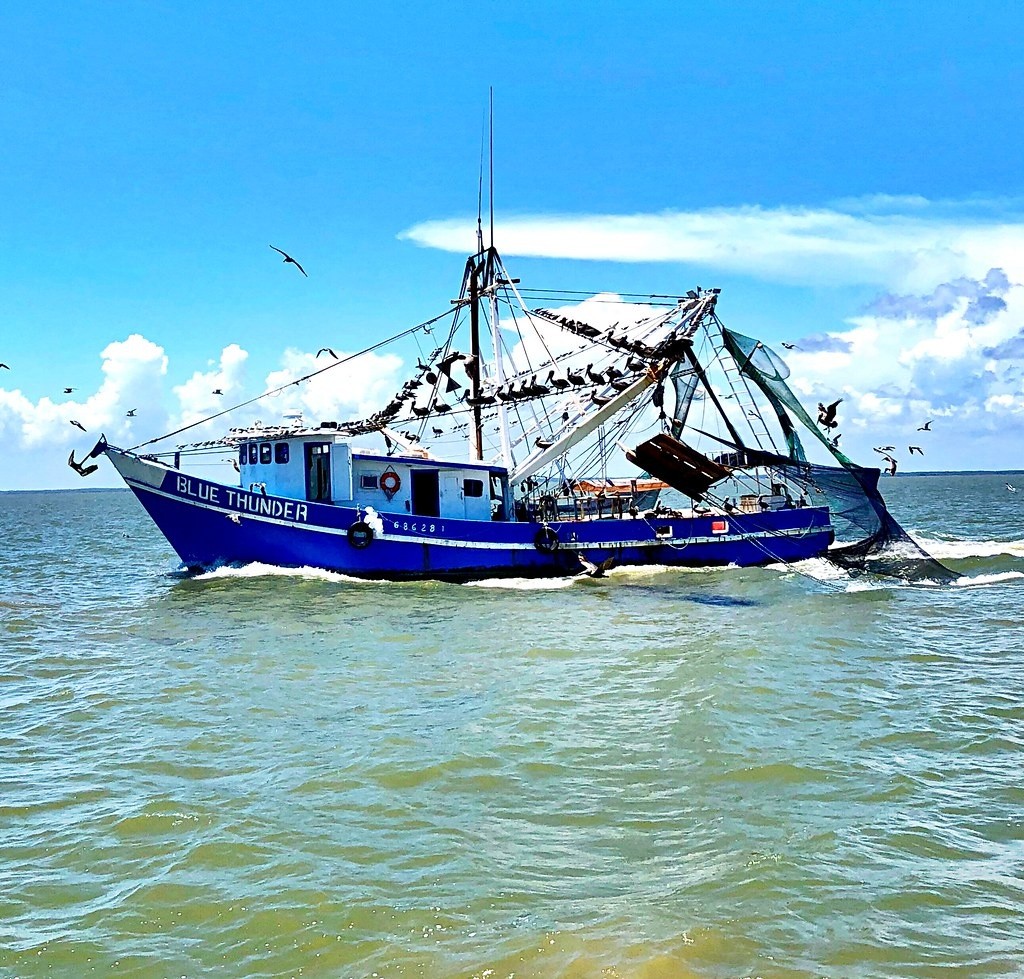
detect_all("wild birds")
[577,552,615,579]
[126,409,137,417]
[316,348,339,359]
[873,446,898,476]
[909,446,923,455]
[0,364,10,370]
[175,426,321,451]
[916,420,933,431]
[70,421,87,432]
[340,306,695,450]
[269,245,308,277]
[64,388,72,393]
[781,342,805,352]
[212,390,223,395]
[816,398,843,448]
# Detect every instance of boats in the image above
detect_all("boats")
[99,87,834,583]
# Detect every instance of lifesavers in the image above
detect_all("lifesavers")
[380,472,401,494]
[532,526,559,554]
[346,522,374,549]
